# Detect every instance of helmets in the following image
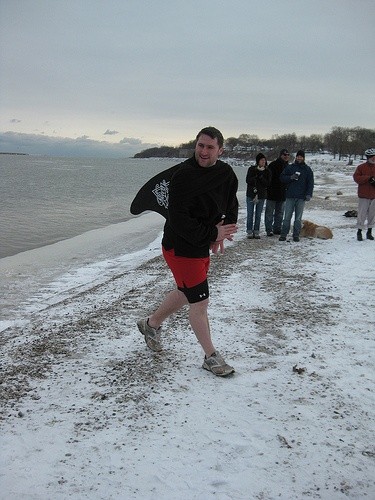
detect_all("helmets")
[365,148,375,156]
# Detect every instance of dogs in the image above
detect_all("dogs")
[294,219,333,240]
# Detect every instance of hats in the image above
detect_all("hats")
[256,153,267,168]
[296,150,304,158]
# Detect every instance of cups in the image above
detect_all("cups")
[295,171,301,180]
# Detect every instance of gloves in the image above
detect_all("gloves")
[306,195,310,201]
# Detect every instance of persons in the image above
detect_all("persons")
[279,150,313,242]
[265,149,290,236]
[353,148,375,241]
[246,153,272,239]
[129,127,240,377]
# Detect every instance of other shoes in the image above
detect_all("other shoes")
[293,236,299,242]
[268,232,273,236]
[247,233,253,238]
[274,230,281,235]
[279,236,286,241]
[253,233,260,239]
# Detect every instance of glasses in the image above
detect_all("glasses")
[283,154,290,156]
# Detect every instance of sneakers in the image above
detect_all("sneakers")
[202,351,234,376]
[136,318,163,351]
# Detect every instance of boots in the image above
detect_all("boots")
[366,228,374,240]
[357,229,362,240]
